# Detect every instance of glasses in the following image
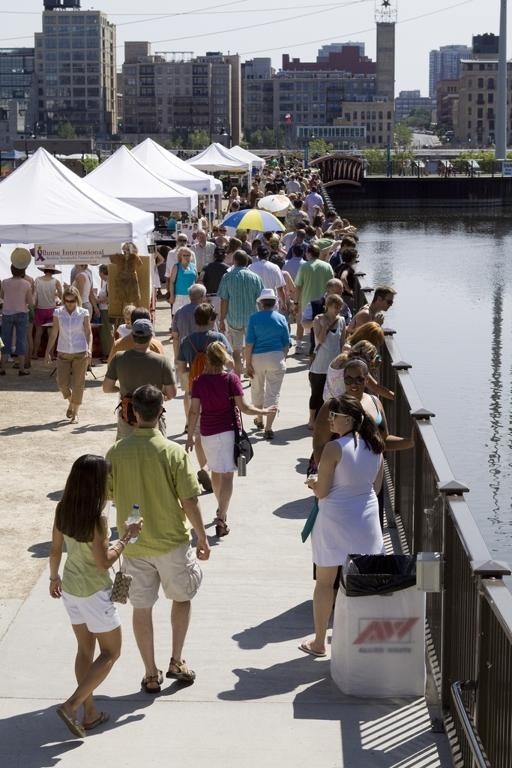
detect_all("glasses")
[65,299,75,303]
[384,298,393,305]
[219,230,226,232]
[344,375,365,386]
[328,411,348,418]
[178,239,186,242]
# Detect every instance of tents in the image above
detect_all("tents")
[0,138,265,289]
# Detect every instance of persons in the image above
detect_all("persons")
[422,160,472,178]
[299,393,384,659]
[175,299,236,496]
[102,382,211,691]
[0,153,417,475]
[185,339,279,538]
[311,358,416,612]
[47,452,144,740]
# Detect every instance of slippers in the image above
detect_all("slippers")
[197,469,213,493]
[298,640,327,656]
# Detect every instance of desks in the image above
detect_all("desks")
[155,239,177,284]
[41,322,104,380]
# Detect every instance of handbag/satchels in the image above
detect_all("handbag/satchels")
[301,502,318,543]
[109,548,133,604]
[233,430,254,466]
[121,394,136,423]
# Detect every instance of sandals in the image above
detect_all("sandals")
[66,409,72,418]
[166,657,196,681]
[141,670,164,693]
[71,413,80,424]
[0,354,56,376]
[215,519,230,537]
[56,701,85,738]
[82,711,110,730]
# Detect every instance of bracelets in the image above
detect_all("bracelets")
[311,480,317,489]
[49,576,60,582]
[118,539,127,549]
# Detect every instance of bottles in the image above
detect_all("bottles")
[238,452,247,476]
[125,505,140,543]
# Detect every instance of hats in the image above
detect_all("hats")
[10,248,31,269]
[132,318,155,337]
[37,265,62,274]
[256,288,279,305]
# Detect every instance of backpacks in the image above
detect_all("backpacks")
[188,330,213,392]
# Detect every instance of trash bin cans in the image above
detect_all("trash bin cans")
[330,553,427,699]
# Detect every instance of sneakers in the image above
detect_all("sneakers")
[294,347,304,354]
[264,430,274,439]
[254,418,264,429]
